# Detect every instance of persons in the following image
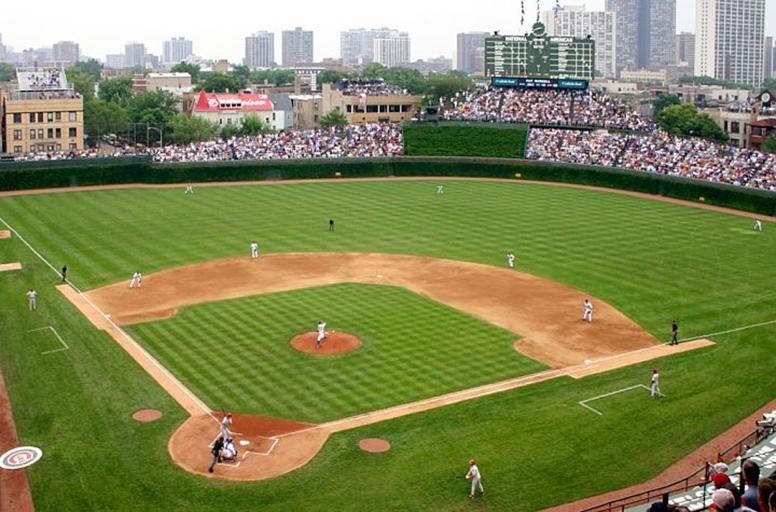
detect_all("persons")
[130,271,142,288]
[507,251,515,268]
[671,320,678,344]
[582,299,593,323]
[208,411,243,472]
[465,459,484,497]
[26,287,38,311]
[753,219,761,231]
[62,264,67,282]
[646,459,776,511]
[316,319,326,349]
[649,368,660,398]
[251,241,259,258]
[329,216,335,231]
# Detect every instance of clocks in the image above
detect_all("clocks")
[531,21,546,37]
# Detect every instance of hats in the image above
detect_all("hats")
[708,461,737,511]
